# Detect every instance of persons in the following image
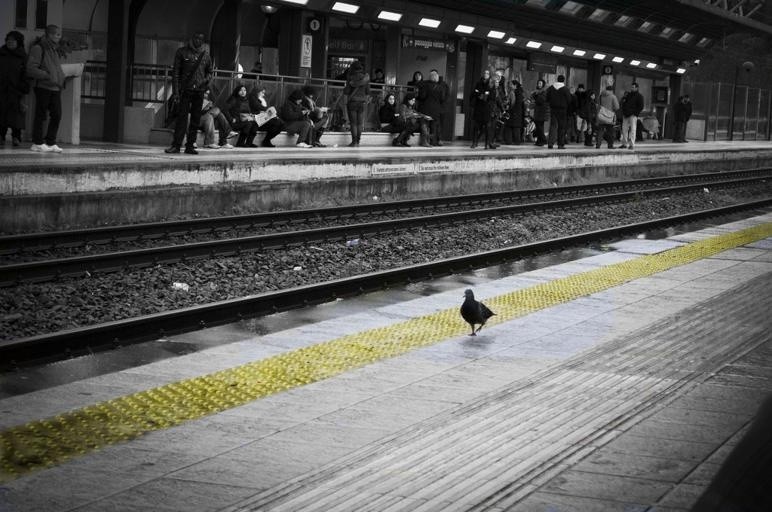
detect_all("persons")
[674,96,686,143]
[0,31,30,147]
[26,24,65,154]
[683,95,692,142]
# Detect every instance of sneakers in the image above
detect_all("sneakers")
[192,142,198,148]
[295,140,327,148]
[203,143,219,149]
[30,142,64,153]
[184,147,198,154]
[219,143,233,148]
[0,136,6,145]
[261,138,275,147]
[470,134,662,149]
[672,137,688,142]
[164,145,180,153]
[12,137,20,146]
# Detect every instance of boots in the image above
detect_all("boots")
[235,124,257,147]
[345,135,361,147]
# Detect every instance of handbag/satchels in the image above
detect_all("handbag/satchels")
[162,93,181,128]
[573,112,587,132]
[523,121,536,135]
[595,106,616,127]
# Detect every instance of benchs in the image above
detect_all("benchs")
[149,125,423,148]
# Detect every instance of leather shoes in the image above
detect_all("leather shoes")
[388,124,446,147]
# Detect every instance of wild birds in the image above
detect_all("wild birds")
[460,286,498,337]
